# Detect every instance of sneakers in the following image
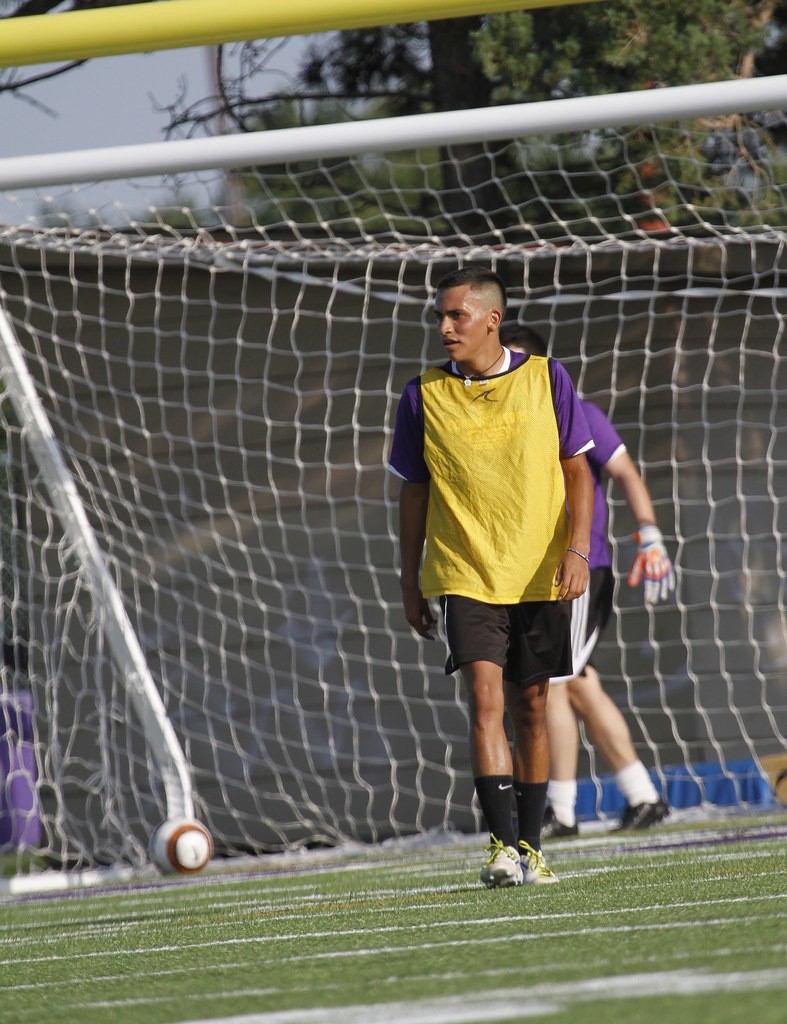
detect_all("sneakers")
[480,832,524,887]
[607,798,674,835]
[518,840,559,885]
[539,815,579,845]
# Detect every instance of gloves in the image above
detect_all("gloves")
[627,526,675,605]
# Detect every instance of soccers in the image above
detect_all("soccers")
[148,815,215,877]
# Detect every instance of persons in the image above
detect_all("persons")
[498,324,676,839]
[384,267,596,889]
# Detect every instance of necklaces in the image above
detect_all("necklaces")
[461,347,504,387]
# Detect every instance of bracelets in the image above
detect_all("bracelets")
[567,548,590,563]
